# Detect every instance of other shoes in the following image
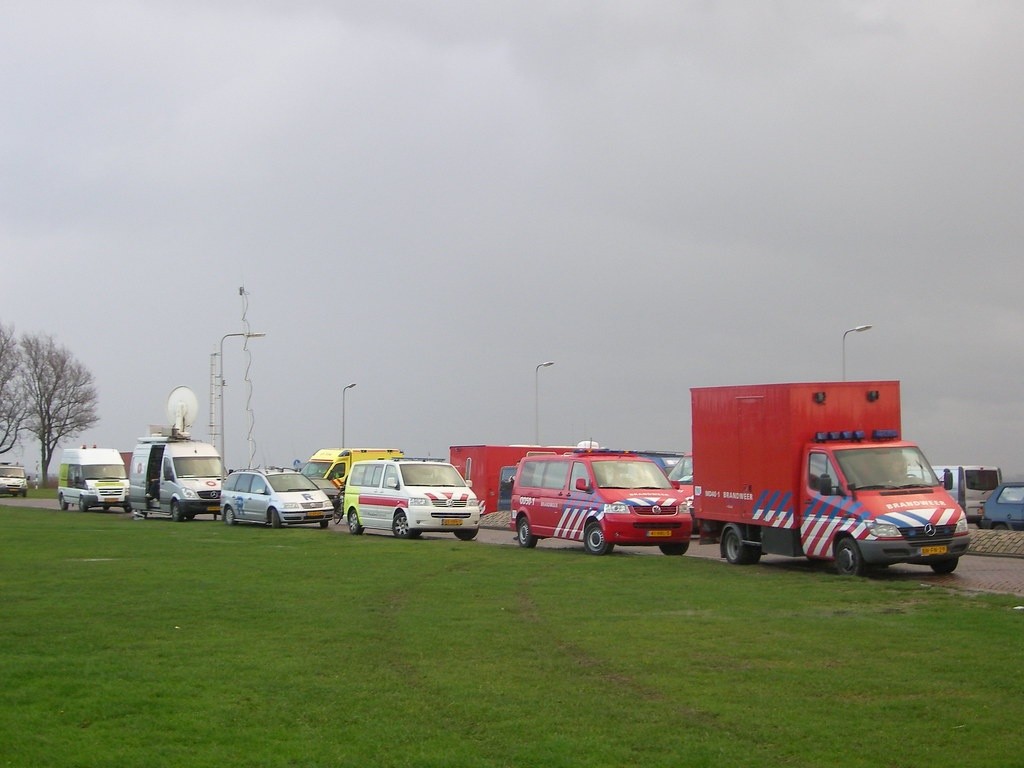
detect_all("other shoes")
[150,498,158,502]
[144,493,153,498]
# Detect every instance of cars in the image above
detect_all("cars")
[310,477,340,508]
[976,481,1022,529]
[219,466,335,531]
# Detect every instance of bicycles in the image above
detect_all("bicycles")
[333,484,347,525]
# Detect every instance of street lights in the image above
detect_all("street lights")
[841,324,871,381]
[532,361,553,448]
[341,383,360,449]
[220,333,265,474]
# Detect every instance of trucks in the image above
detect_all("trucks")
[450,445,609,516]
[691,379,973,582]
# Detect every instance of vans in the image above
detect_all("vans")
[128,434,229,520]
[0,463,30,498]
[300,449,404,496]
[58,444,133,513]
[347,459,480,539]
[669,452,701,534]
[510,451,691,557]
[907,466,1003,528]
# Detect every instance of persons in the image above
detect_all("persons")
[876,454,916,486]
[143,459,161,502]
